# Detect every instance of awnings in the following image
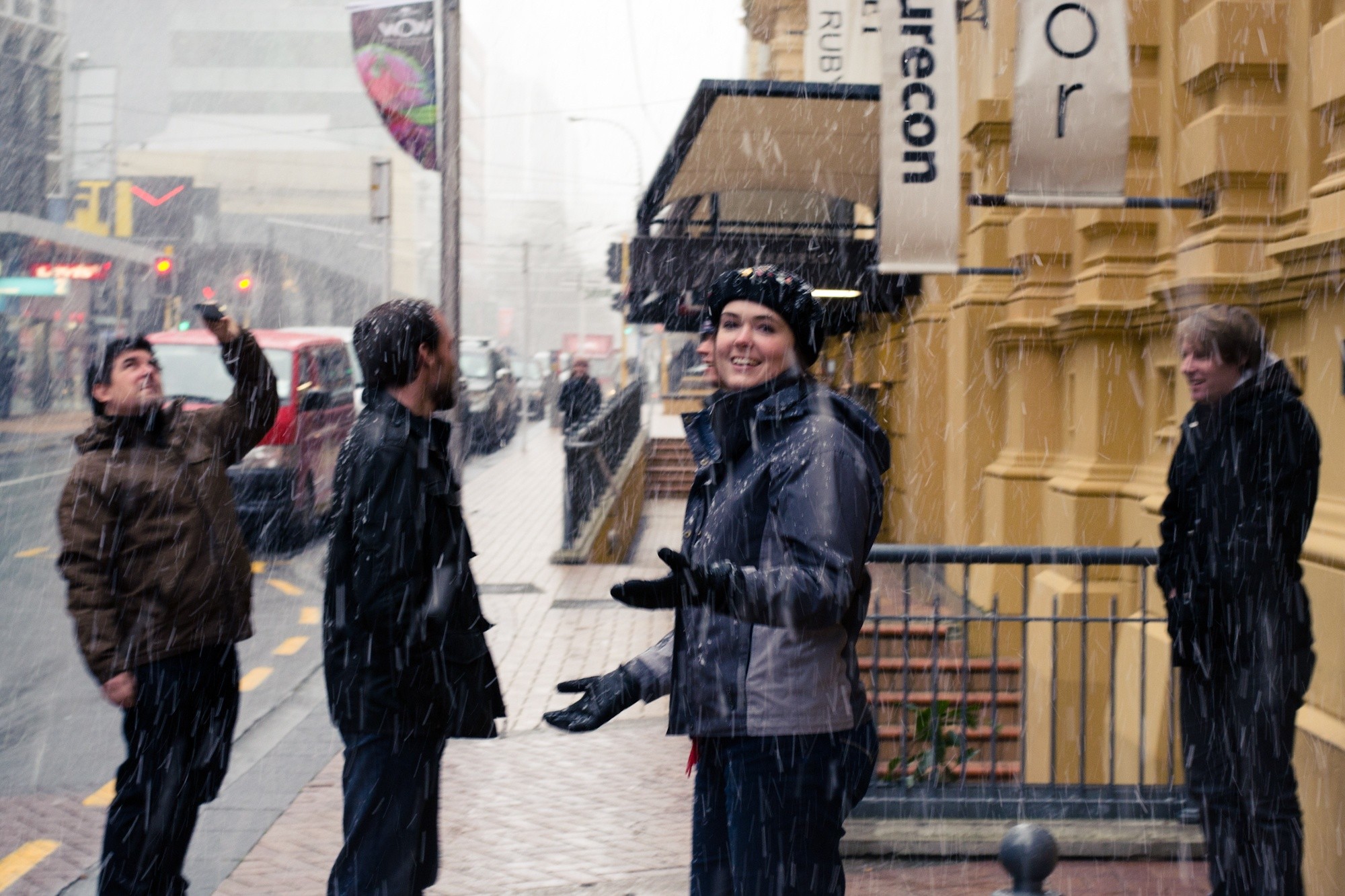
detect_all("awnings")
[605,78,920,337]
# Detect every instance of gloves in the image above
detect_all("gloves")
[609,547,707,610]
[542,666,640,733]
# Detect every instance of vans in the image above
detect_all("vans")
[143,333,356,556]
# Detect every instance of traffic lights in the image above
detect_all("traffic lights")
[236,274,256,306]
[150,255,176,300]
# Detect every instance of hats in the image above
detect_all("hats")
[707,263,823,368]
[573,357,589,367]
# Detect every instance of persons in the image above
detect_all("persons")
[0,313,109,419]
[1155,303,1321,896]
[54,300,280,896]
[610,264,891,896]
[323,299,506,896]
[541,314,879,828]
[557,359,601,437]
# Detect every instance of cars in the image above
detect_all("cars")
[454,335,574,451]
